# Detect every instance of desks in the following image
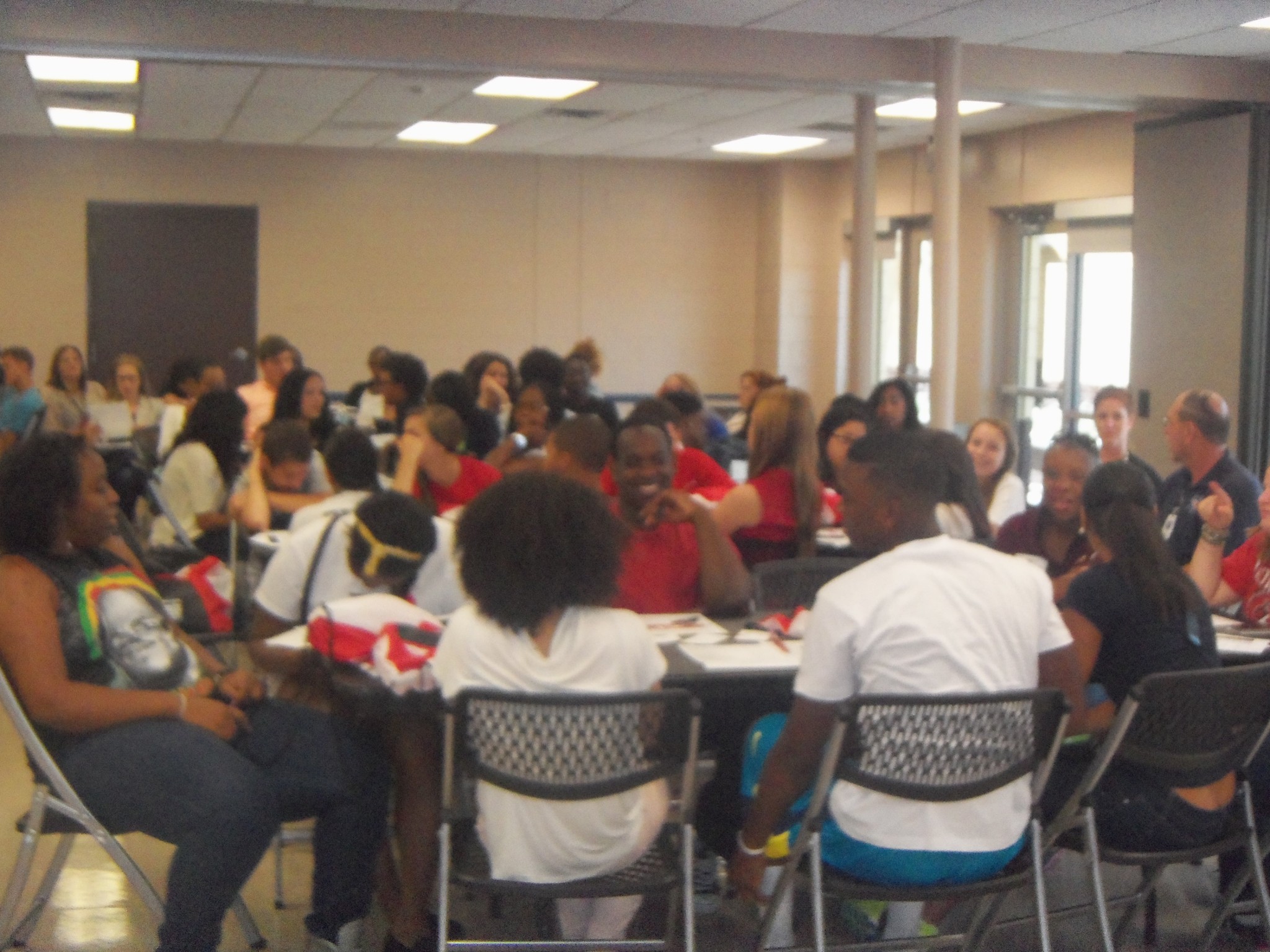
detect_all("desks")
[317,609,1270,952]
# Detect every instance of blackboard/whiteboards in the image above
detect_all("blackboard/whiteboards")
[84,200,260,395]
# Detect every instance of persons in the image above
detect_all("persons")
[1087,385,1164,486]
[1040,460,1239,856]
[164,336,928,713]
[81,353,165,452]
[1160,386,1270,934]
[0,346,44,455]
[39,345,109,442]
[129,385,267,574]
[248,491,473,952]
[0,432,390,952]
[427,468,670,940]
[961,414,1024,528]
[605,424,760,897]
[995,431,1114,736]
[734,426,1072,946]
[229,419,334,534]
[393,402,502,520]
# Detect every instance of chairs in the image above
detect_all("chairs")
[0,426,1270,952]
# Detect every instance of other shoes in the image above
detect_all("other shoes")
[843,897,939,939]
[305,909,365,952]
[384,914,463,952]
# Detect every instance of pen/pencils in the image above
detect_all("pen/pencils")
[773,634,791,654]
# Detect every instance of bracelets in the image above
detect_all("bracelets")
[172,689,188,721]
[210,668,231,678]
[734,830,765,857]
[1200,522,1231,544]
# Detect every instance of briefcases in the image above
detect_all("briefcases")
[693,858,721,916]
